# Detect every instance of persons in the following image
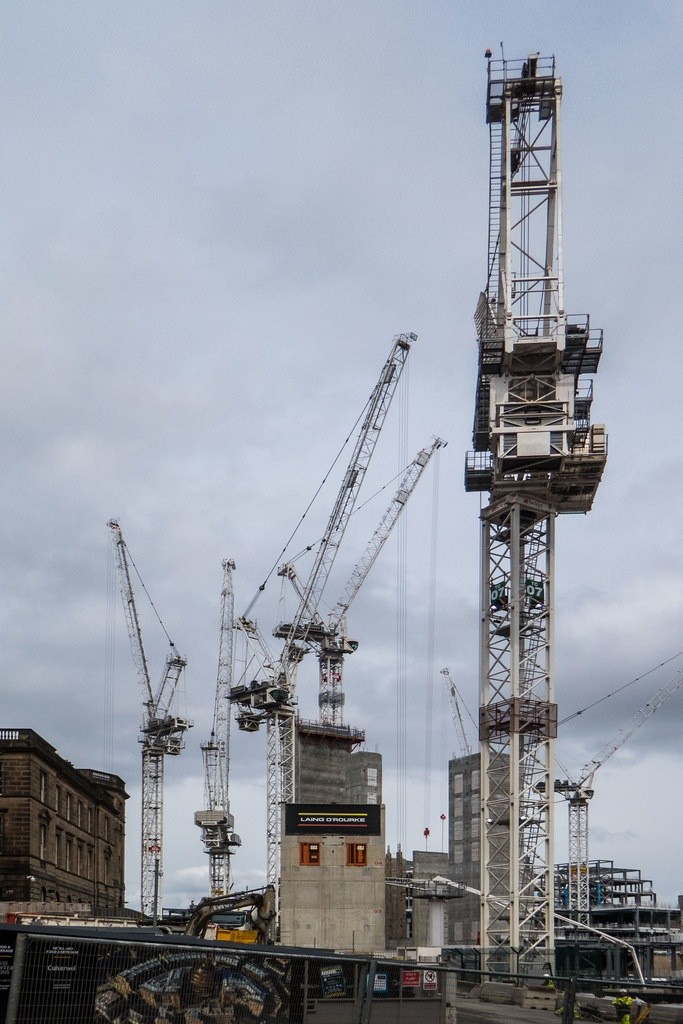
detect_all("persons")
[554,989,584,1021]
[612,988,633,1024]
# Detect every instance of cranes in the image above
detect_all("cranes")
[230,323,448,951]
[465,41,618,999]
[97,510,187,931]
[196,556,241,893]
